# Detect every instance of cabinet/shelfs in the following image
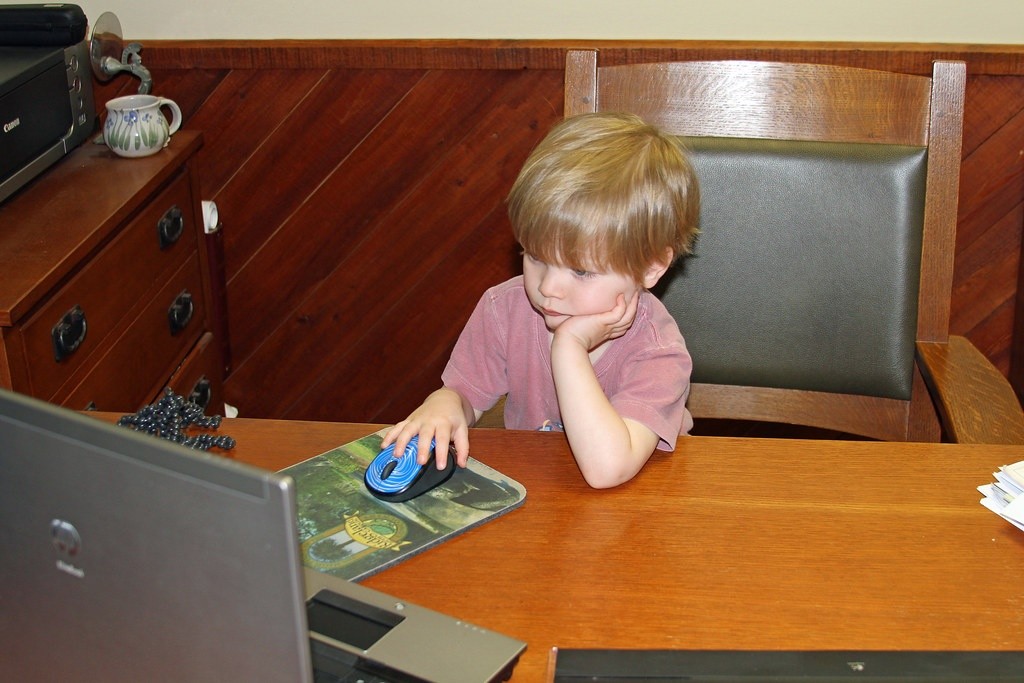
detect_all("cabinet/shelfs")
[0,118,230,418]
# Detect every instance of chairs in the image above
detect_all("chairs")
[561,47,1023,443]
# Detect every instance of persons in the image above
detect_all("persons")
[380,112,703,492]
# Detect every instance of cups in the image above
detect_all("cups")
[103,95,182,158]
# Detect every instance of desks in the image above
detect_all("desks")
[2,399,1023,683]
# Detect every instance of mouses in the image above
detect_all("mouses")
[364,434,454,503]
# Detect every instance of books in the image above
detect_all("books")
[975,458,1024,534]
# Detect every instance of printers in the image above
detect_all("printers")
[1,38,100,206]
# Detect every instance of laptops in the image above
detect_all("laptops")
[0,392,528,683]
